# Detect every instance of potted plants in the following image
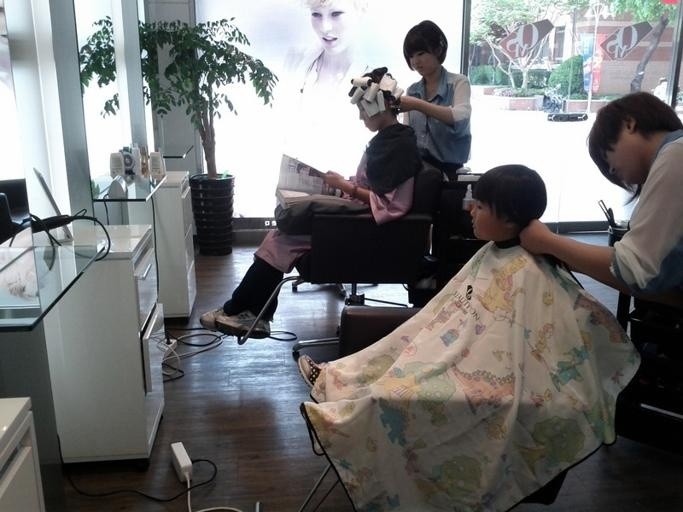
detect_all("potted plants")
[138,16,280,255]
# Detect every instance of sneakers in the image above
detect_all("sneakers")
[296,354,322,387]
[199,306,227,332]
[215,311,269,339]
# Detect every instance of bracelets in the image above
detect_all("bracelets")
[352,184,358,198]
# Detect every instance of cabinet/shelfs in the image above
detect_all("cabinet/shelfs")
[127,171,198,324]
[36,224,166,474]
[1,397,48,512]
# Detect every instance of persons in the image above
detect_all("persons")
[302,0,370,85]
[199,65,425,338]
[395,19,473,308]
[516,89,683,299]
[296,165,547,388]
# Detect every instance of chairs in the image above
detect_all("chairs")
[292,168,442,356]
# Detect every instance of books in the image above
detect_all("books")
[275,153,352,210]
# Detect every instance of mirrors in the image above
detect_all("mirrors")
[74,0,134,199]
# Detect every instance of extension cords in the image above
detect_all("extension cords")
[163,337,178,355]
[169,441,194,483]
